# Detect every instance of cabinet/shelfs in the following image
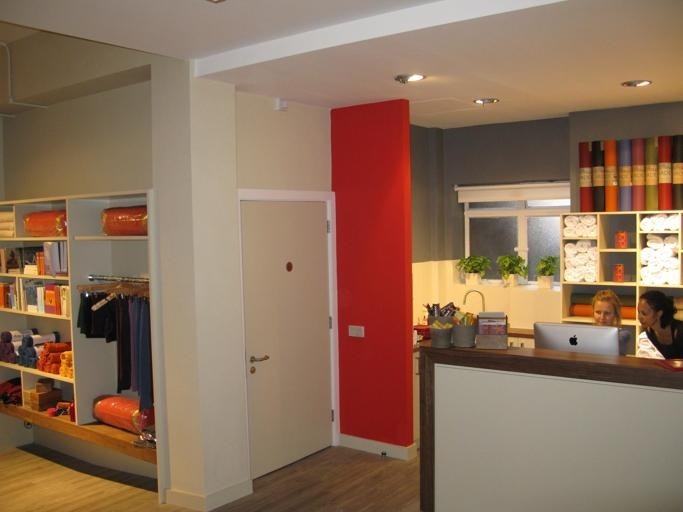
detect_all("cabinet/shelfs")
[0,199,66,432]
[66,187,154,470]
[560,211,682,356]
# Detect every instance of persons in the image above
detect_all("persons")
[636,291,682,359]
[590,289,632,356]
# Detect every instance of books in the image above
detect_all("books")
[0,241,68,318]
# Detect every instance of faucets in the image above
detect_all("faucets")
[463,289,486,312]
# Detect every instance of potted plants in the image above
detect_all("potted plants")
[537,252,559,290]
[455,253,491,284]
[496,254,529,286]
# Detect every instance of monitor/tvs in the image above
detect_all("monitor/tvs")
[533,321,620,357]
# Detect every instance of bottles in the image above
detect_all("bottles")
[423,302,477,328]
[69,398,74,422]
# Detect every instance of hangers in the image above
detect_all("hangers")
[77,275,149,310]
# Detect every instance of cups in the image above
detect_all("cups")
[450,325,476,348]
[428,327,452,349]
[46,408,55,416]
